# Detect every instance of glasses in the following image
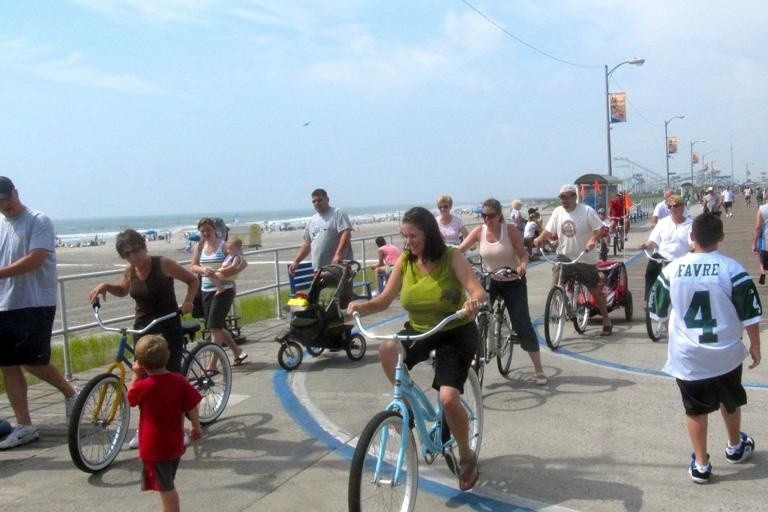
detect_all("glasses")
[439,205,449,209]
[121,245,144,259]
[481,212,500,219]
[668,204,680,209]
[311,198,324,203]
[559,195,574,200]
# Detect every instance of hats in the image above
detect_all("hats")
[708,186,713,190]
[528,208,539,213]
[0,175,15,201]
[560,184,577,193]
[598,207,605,214]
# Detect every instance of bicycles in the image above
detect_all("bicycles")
[609,213,633,251]
[463,264,514,389]
[67,292,234,476]
[640,251,691,342]
[597,226,611,260]
[530,242,591,352]
[343,304,485,509]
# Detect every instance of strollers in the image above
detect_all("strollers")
[275,258,367,373]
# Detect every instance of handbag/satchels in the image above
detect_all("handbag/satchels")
[191,274,204,318]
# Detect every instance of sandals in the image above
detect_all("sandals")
[458,450,480,491]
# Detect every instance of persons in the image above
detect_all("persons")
[640,196,694,311]
[371,236,403,297]
[608,191,627,249]
[126,334,203,512]
[0,176,82,450]
[288,189,353,351]
[214,236,247,297]
[533,185,613,337]
[346,207,488,491]
[648,215,763,484]
[431,194,477,251]
[141,231,172,243]
[597,208,611,249]
[457,199,547,384]
[262,221,307,233]
[510,200,558,261]
[650,182,768,227]
[54,235,106,248]
[89,229,200,449]
[191,218,248,378]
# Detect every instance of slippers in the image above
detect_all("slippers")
[202,368,220,378]
[232,352,248,367]
[600,319,613,337]
[759,273,765,285]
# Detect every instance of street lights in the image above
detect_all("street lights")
[745,161,754,182]
[603,58,646,174]
[701,149,722,181]
[688,138,706,183]
[663,114,684,191]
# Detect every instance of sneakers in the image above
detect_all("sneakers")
[534,372,547,385]
[0,424,41,450]
[724,431,755,464]
[65,386,80,418]
[687,451,712,484]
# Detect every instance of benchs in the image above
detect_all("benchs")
[288,263,372,309]
[378,272,394,294]
